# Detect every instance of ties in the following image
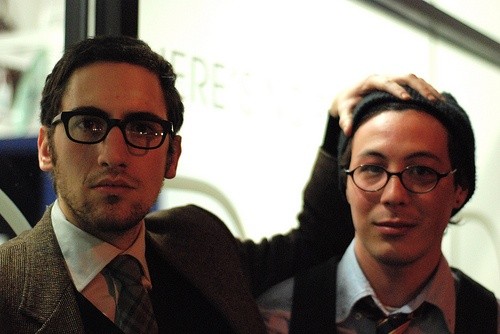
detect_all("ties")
[354,296,414,334]
[100,255,158,334]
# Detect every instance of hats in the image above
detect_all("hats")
[349,88,476,217]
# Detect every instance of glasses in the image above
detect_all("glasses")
[344,165,454,194]
[51,112,174,149]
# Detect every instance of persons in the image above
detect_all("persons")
[0,33,447,334]
[289,87,499,334]
